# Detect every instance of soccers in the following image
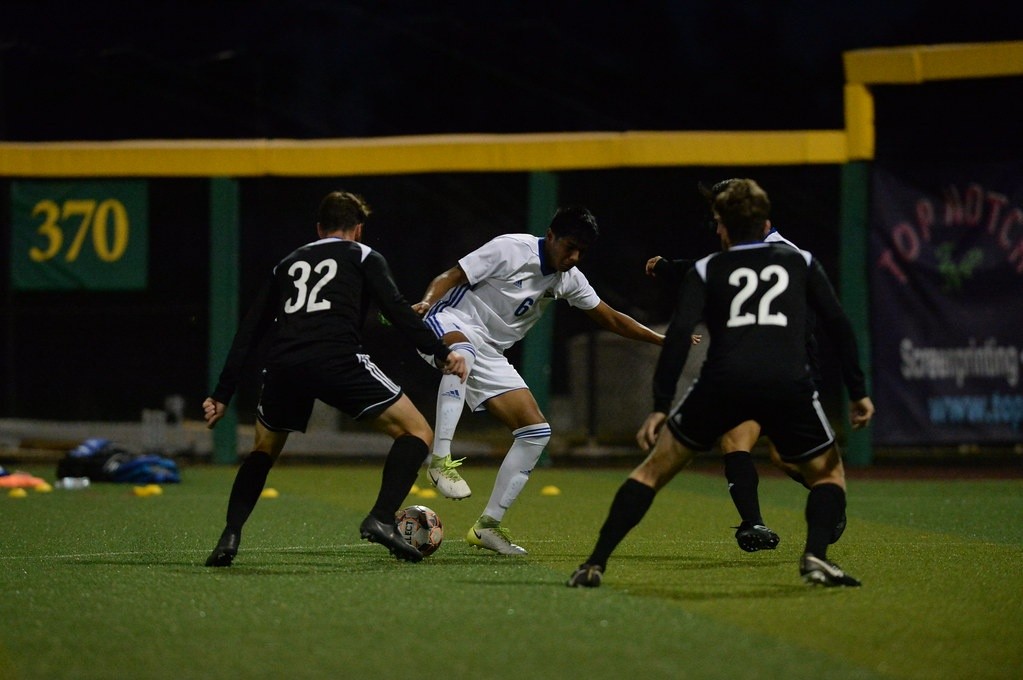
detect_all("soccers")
[392,504,443,557]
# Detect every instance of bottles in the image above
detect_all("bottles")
[54,477,89,489]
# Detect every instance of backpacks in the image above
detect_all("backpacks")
[56,438,180,485]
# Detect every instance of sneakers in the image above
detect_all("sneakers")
[731,521,780,552]
[427,453,471,500]
[565,564,605,587]
[798,553,862,588]
[467,521,527,555]
[205,531,239,566]
[359,512,423,563]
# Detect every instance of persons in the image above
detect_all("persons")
[408,202,703,558]
[201,189,468,568]
[563,176,875,589]
